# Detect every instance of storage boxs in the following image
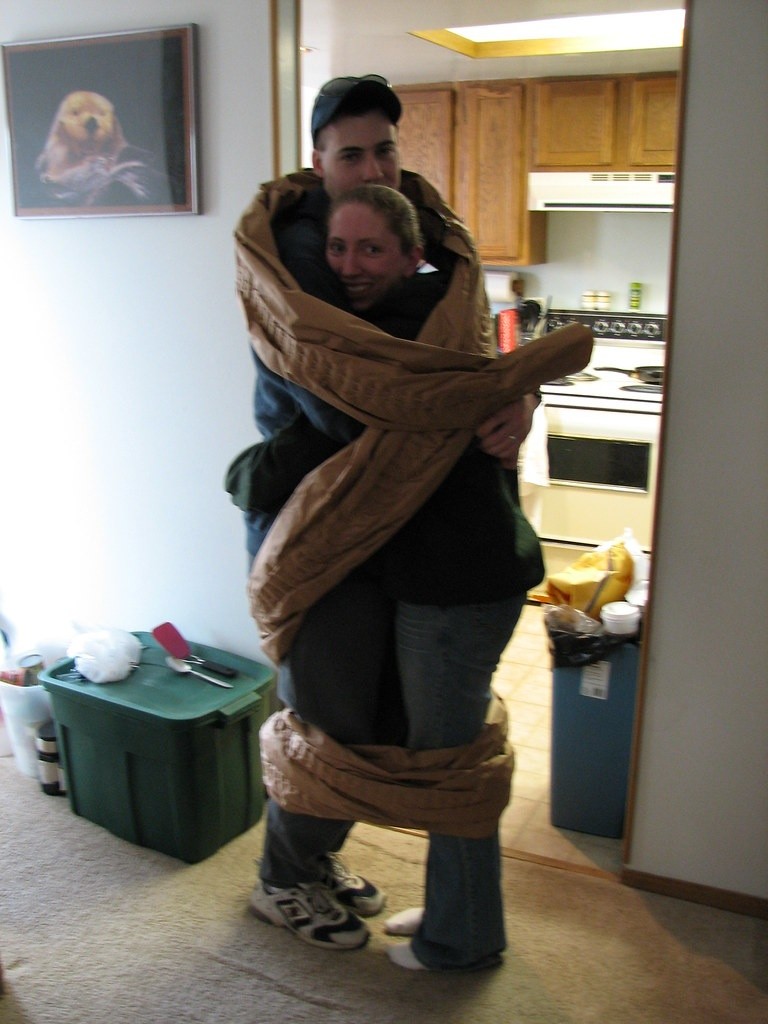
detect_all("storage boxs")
[37,632,279,868]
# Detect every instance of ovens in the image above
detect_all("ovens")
[528,398,661,552]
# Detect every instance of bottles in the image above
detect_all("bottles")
[36,724,60,795]
[630,283,641,308]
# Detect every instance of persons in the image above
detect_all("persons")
[225,185,546,974]
[250,74,542,950]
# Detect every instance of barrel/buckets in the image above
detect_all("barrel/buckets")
[0,646,73,778]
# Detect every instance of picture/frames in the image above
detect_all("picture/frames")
[0,22,204,221]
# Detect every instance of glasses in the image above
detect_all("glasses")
[313,74,393,111]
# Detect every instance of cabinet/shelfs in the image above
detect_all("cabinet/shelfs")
[528,70,681,173]
[391,77,549,266]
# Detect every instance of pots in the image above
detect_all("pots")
[594,363,664,383]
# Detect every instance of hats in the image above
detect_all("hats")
[311,82,402,142]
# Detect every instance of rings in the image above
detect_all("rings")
[509,436,516,439]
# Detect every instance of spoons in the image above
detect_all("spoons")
[165,656,234,687]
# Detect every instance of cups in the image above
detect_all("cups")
[582,291,596,309]
[599,601,640,634]
[596,290,612,309]
[498,309,521,354]
[623,581,648,615]
[522,298,545,340]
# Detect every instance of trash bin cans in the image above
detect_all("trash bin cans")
[0,680,52,778]
[542,610,648,840]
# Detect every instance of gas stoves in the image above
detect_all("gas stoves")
[538,357,666,400]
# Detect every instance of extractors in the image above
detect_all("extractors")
[527,171,677,213]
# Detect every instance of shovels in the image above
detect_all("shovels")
[152,622,238,679]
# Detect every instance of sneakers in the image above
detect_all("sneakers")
[249,879,370,952]
[320,851,386,915]
